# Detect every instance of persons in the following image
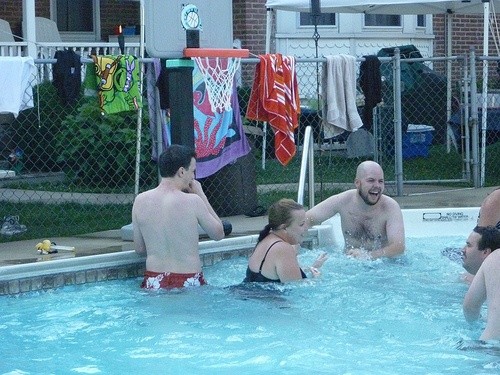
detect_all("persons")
[238,197,329,288]
[132,145,225,293]
[461,249,500,346]
[299,160,405,261]
[458,227,500,286]
[476,187,500,230]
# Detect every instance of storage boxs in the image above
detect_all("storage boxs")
[402,124,435,161]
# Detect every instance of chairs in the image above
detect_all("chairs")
[22,16,66,60]
[0,19,17,57]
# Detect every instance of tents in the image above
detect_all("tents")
[261,0,500,185]
